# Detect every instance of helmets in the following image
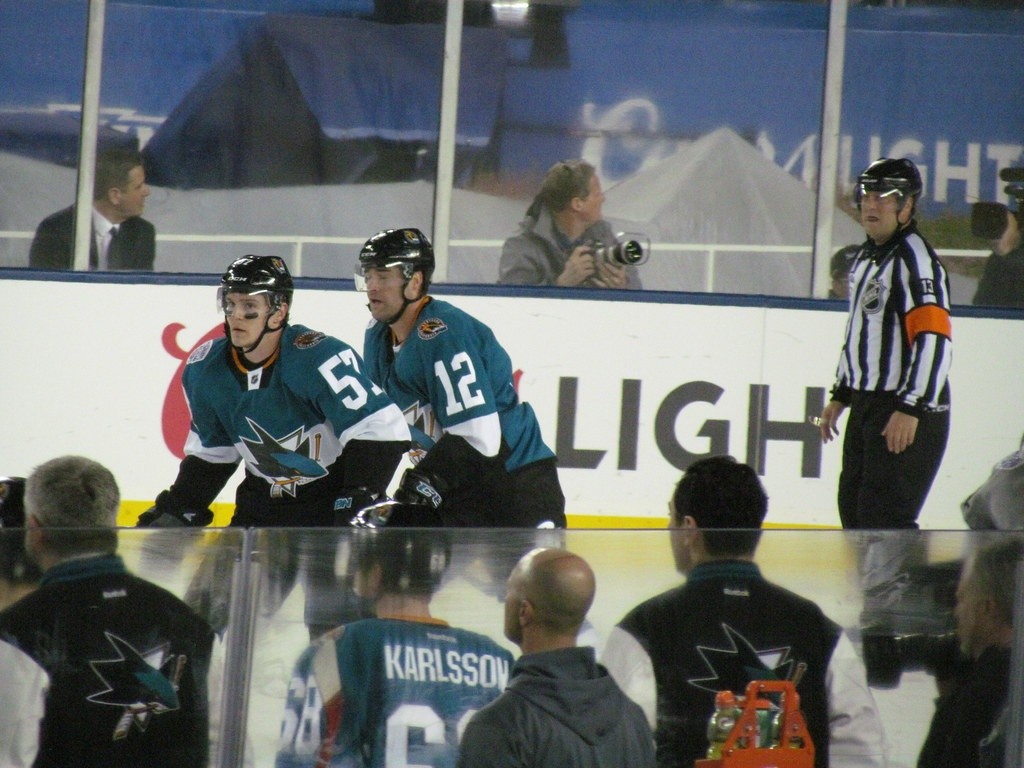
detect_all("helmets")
[349,501,452,584]
[857,158,923,210]
[0,476,38,583]
[359,228,435,279]
[220,255,293,309]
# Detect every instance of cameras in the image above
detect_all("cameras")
[582,240,644,278]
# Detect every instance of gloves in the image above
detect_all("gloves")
[334,489,381,527]
[392,468,445,508]
[135,486,214,570]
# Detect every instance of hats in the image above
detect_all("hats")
[831,245,860,278]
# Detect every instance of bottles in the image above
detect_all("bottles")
[706,691,802,760]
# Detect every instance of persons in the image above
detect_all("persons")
[0,476,45,608]
[0,455,215,768]
[457,546,657,768]
[912,534,1024,768]
[495,160,643,289]
[827,244,858,301]
[362,229,607,659]
[818,159,952,690]
[274,502,517,768]
[971,211,1024,308]
[132,257,412,644]
[595,455,892,768]
[29,144,156,272]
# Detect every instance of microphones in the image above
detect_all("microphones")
[1000,168,1024,183]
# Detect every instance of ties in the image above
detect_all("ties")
[106,227,120,271]
